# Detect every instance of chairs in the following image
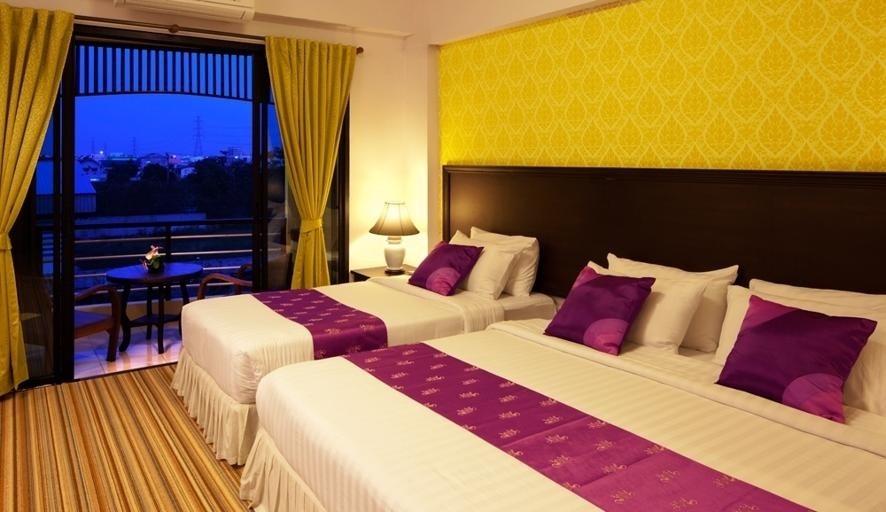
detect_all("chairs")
[49,284,122,362]
[197,254,291,301]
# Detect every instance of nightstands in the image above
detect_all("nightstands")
[351,264,419,282]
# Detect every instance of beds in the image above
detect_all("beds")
[238,318,886,512]
[169,274,566,466]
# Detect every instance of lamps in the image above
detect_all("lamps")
[369,200,419,274]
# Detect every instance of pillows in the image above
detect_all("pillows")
[407,226,541,297]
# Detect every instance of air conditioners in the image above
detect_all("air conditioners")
[114,0,255,25]
